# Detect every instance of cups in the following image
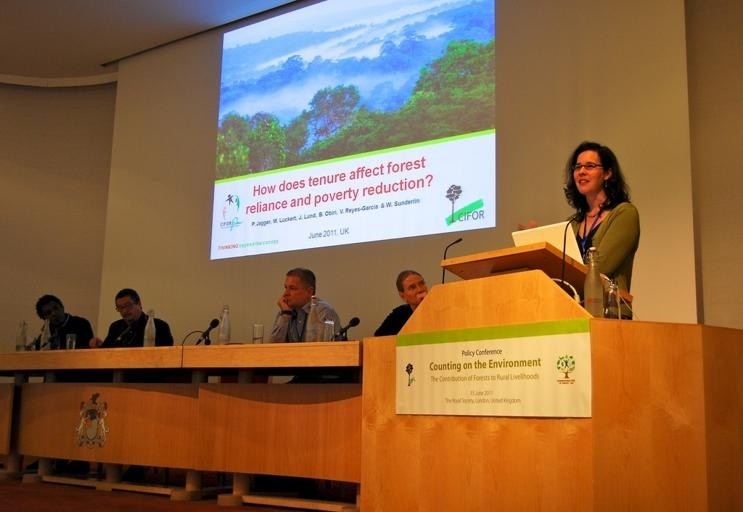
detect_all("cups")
[251,322,264,344]
[65,333,76,349]
[321,319,335,342]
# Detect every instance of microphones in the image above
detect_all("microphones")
[334,318,360,336]
[196,319,219,344]
[441,238,462,283]
[561,212,580,289]
[118,328,134,342]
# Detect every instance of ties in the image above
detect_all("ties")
[296,308,305,342]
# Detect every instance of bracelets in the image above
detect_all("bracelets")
[282,311,293,315]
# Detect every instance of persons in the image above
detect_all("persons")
[517,143,640,320]
[25,295,94,476]
[88,289,174,483]
[253,268,352,499]
[375,271,429,336]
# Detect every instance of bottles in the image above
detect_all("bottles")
[142,309,155,347]
[217,305,230,346]
[583,246,604,319]
[303,294,319,342]
[15,318,27,351]
[604,279,621,319]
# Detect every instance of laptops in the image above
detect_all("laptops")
[511,222,611,281]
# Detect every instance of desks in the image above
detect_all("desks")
[0,335,234,503]
[182,339,362,511]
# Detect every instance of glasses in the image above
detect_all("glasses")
[113,300,138,313]
[570,161,604,171]
